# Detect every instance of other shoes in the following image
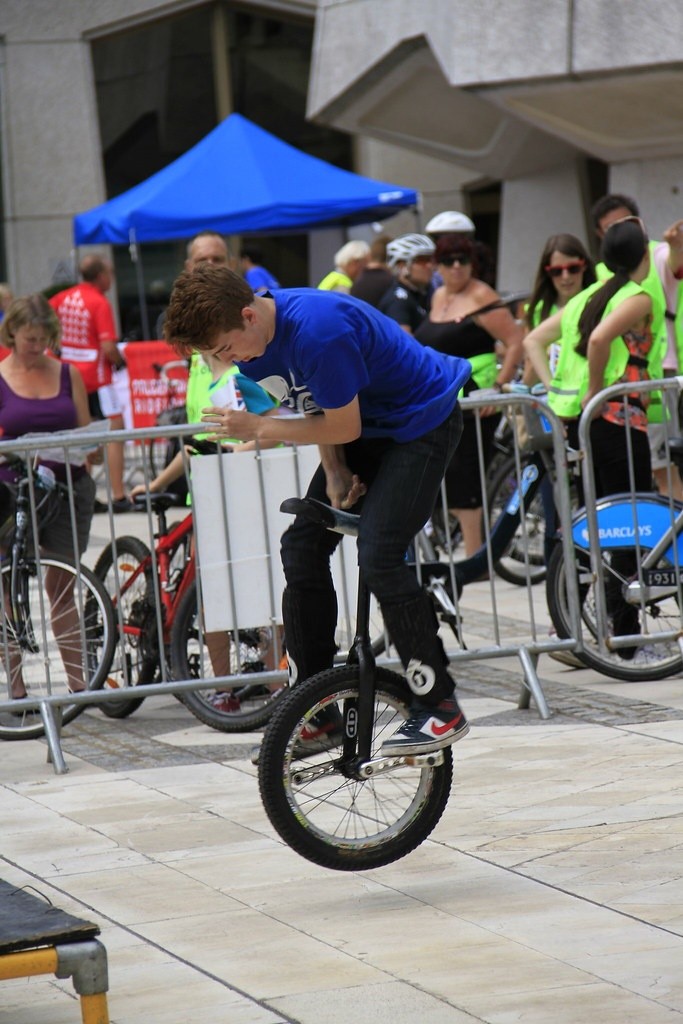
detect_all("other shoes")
[10,692,33,716]
[113,496,145,512]
[547,636,592,670]
[608,597,642,661]
[92,499,109,515]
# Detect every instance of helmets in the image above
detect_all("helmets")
[424,210,477,233]
[387,231,437,261]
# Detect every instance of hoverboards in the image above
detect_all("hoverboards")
[258,497,454,871]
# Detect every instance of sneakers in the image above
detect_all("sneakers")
[381,694,470,756]
[207,691,239,713]
[252,699,345,766]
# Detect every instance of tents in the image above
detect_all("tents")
[70,111,425,341]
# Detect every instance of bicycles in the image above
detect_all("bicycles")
[408,381,683,682]
[0,450,117,742]
[83,435,291,733]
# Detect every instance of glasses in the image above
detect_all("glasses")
[608,215,647,239]
[439,253,469,266]
[544,258,585,276]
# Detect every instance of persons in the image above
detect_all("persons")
[126,342,290,717]
[321,192,683,666]
[0,293,104,717]
[44,253,126,424]
[154,268,473,760]
[156,229,280,445]
